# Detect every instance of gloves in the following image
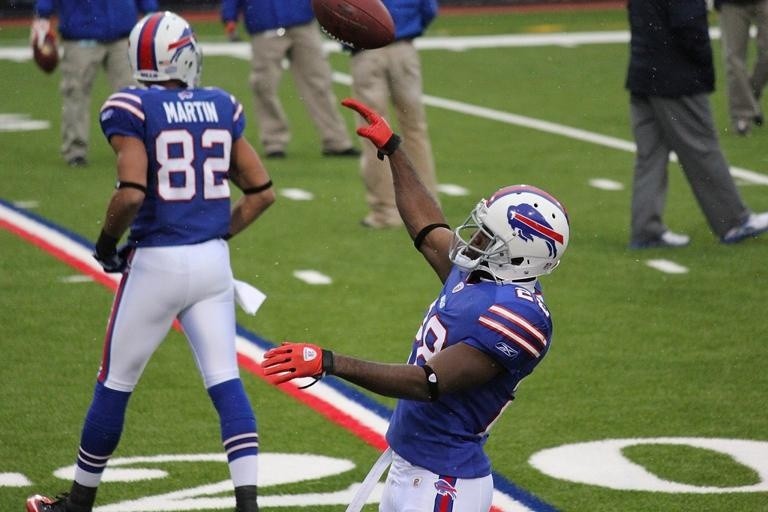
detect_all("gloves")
[30,17,63,51]
[261,341,331,385]
[340,100,400,157]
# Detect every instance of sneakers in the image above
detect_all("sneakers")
[734,107,764,133]
[721,208,767,241]
[362,215,390,232]
[24,492,89,511]
[630,229,690,248]
[263,148,360,159]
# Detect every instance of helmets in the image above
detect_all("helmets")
[449,182,568,282]
[128,10,202,90]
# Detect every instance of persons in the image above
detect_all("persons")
[30,1,165,167]
[344,1,442,232]
[705,0,768,137]
[220,1,360,159]
[620,0,768,252]
[24,10,276,511]
[263,102,572,512]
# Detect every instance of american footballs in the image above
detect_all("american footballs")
[312,0,397,50]
